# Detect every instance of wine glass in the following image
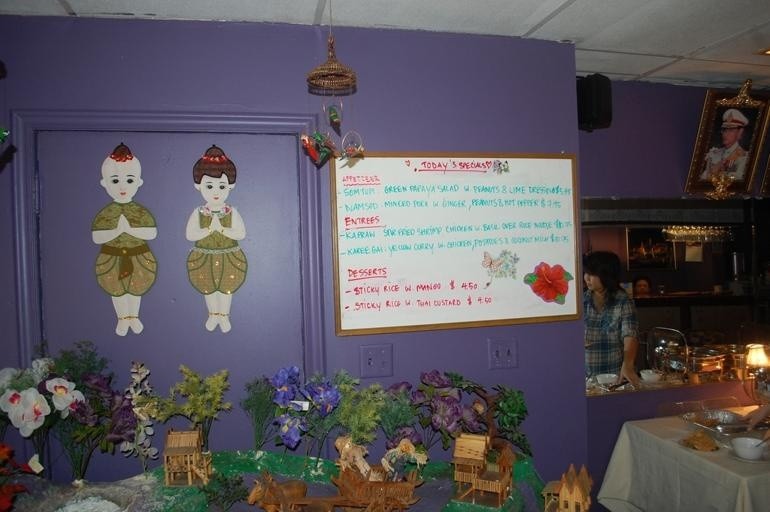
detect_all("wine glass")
[660,224,735,243]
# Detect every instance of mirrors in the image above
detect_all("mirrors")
[625,225,742,297]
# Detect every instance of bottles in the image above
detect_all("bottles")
[637,242,646,262]
[644,237,655,260]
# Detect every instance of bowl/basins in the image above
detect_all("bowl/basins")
[641,369,664,384]
[693,344,746,373]
[595,373,619,386]
[730,436,768,462]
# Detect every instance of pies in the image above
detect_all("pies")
[679,430,718,451]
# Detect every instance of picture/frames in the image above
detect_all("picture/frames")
[681,89,770,200]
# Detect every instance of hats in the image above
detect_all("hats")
[720,109,749,129]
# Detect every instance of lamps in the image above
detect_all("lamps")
[305,1,356,97]
[663,226,730,247]
[731,343,770,402]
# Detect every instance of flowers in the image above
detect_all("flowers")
[1,443,43,512]
[238,366,482,471]
[0,342,233,482]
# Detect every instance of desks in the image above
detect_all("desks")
[594,403,768,511]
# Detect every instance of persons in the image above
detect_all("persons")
[89,140,158,337]
[635,276,653,299]
[699,108,751,182]
[183,142,250,334]
[741,400,769,443]
[582,251,642,391]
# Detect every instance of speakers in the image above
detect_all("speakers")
[577,73,612,132]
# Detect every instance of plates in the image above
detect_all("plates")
[677,437,724,456]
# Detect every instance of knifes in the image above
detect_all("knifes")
[607,381,629,392]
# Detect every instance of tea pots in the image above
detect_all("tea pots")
[649,326,691,374]
